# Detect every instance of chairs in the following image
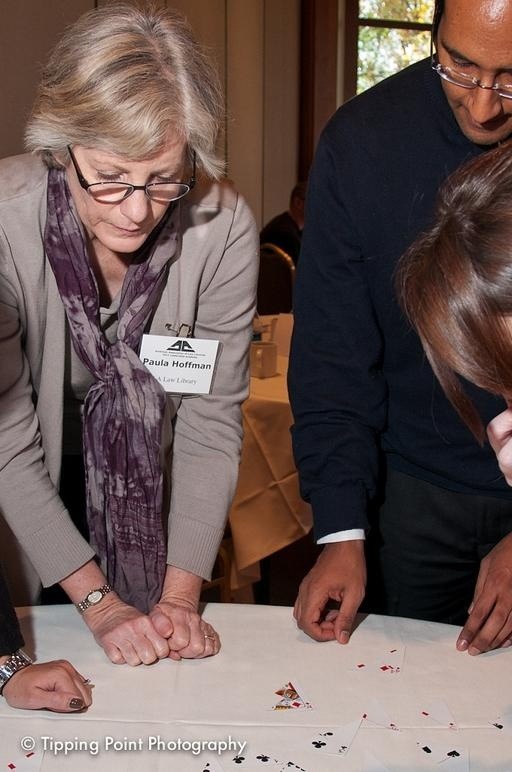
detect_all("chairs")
[256,242,296,315]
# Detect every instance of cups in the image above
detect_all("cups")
[249,342,278,377]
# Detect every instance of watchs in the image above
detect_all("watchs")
[75,583,116,618]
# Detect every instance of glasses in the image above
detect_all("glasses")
[430,34,512,101]
[67,144,197,204]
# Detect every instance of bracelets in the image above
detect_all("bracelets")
[2,648,34,697]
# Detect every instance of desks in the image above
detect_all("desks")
[207,310,316,600]
[2,600,510,772]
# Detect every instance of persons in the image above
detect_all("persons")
[257,180,305,313]
[0,532,94,715]
[3,2,265,669]
[285,0,512,654]
[390,128,512,498]
